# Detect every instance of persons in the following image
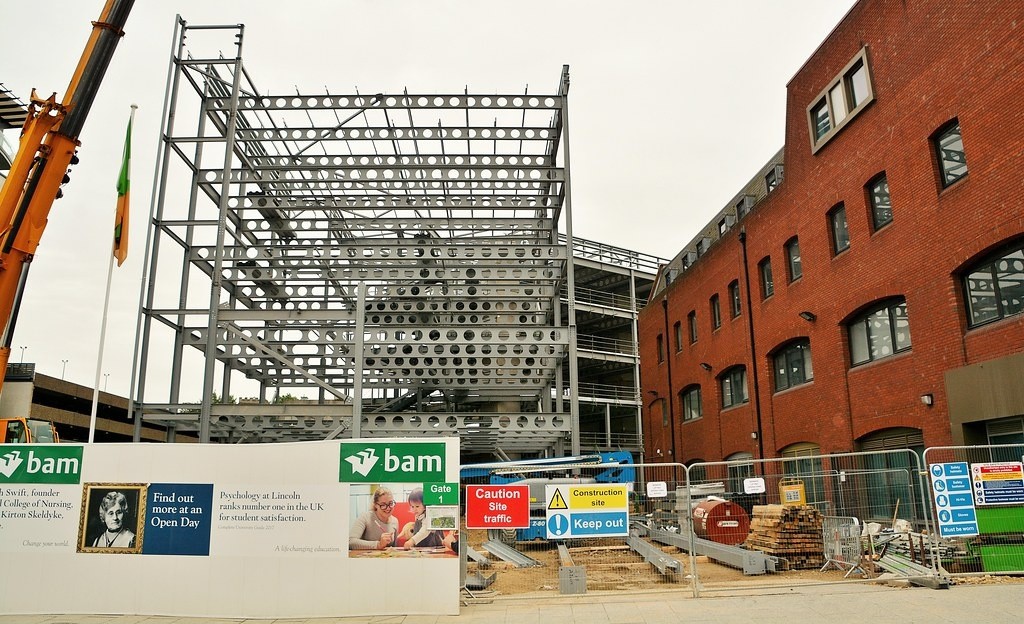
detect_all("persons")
[442,533,458,555]
[404,487,444,551]
[348,486,398,550]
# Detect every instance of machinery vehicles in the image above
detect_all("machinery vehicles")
[457,450,637,553]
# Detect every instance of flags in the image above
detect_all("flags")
[112,116,132,267]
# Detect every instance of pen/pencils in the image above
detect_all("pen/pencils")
[375,520,386,534]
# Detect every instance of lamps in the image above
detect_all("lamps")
[920,393,933,406]
[648,390,659,398]
[799,310,819,324]
[751,431,758,440]
[700,362,712,371]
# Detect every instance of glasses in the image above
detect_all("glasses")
[376,500,397,510]
